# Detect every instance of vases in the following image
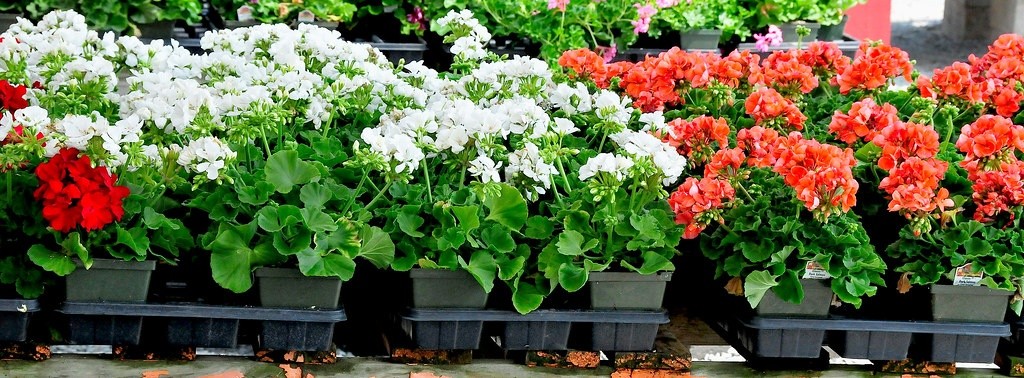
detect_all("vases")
[355,31,427,73]
[134,17,176,39]
[929,279,1015,322]
[756,277,834,315]
[587,269,675,310]
[817,15,848,42]
[291,19,339,32]
[681,29,722,50]
[225,18,263,31]
[409,265,490,307]
[615,47,662,65]
[780,21,821,43]
[64,257,156,303]
[254,265,342,311]
[737,43,773,68]
[0,13,20,34]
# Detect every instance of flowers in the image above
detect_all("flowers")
[0,0,1024,318]
